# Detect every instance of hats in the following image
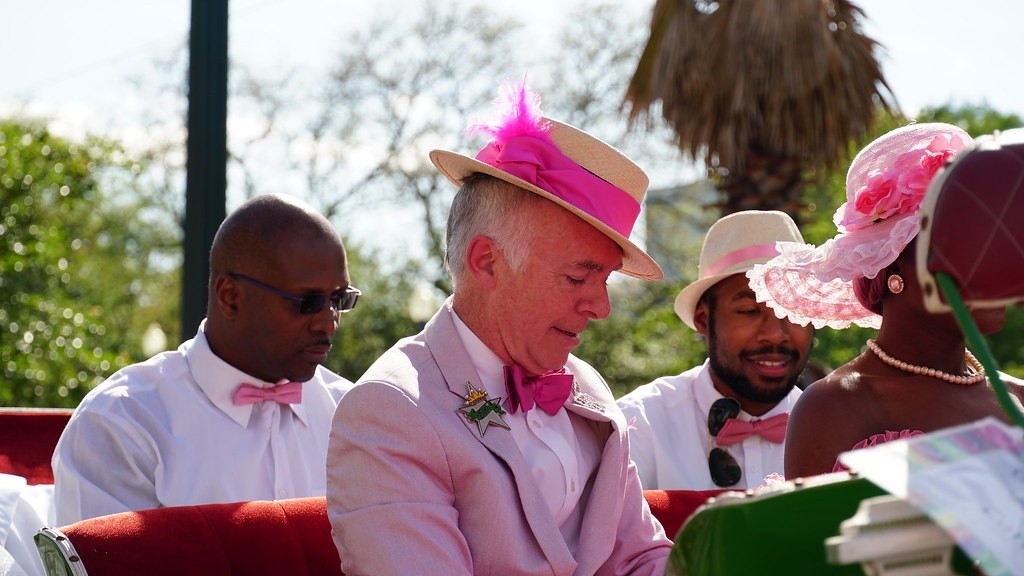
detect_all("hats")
[675,210,805,330]
[430,72,664,282]
[744,123,975,330]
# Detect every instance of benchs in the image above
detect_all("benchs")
[34,488,749,576]
[0,407,74,485]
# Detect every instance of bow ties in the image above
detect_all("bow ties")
[503,364,575,417]
[232,381,302,405]
[716,411,790,448]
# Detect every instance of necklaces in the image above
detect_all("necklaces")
[867,340,985,383]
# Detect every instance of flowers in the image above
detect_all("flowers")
[854,131,959,224]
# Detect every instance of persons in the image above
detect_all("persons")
[51,192,354,526]
[614,210,815,491]
[747,122,1024,481]
[326,78,674,575]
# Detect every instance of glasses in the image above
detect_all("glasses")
[708,397,743,487]
[224,271,362,316]
